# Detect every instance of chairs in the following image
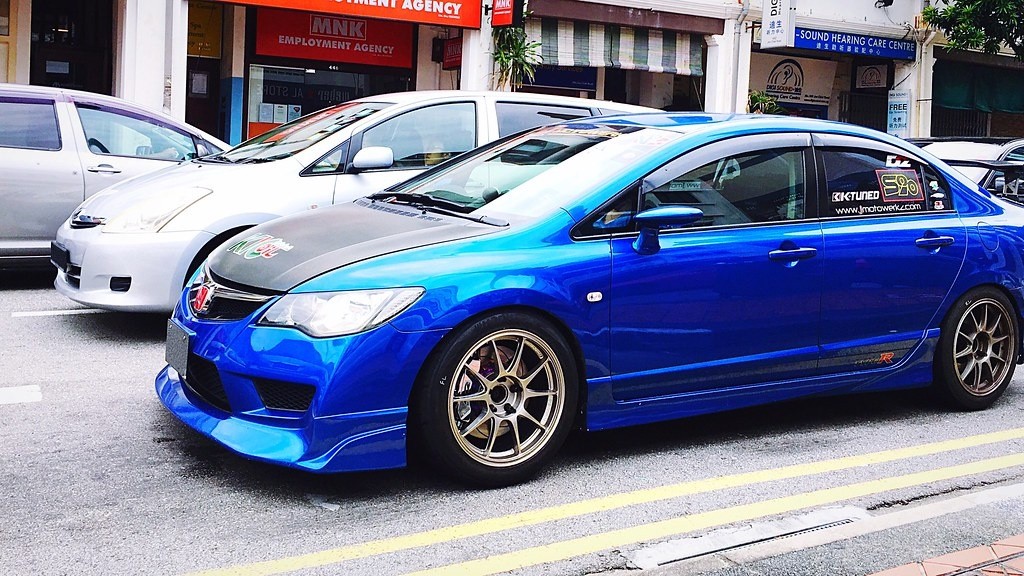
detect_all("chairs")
[387,129,424,168]
[441,129,474,159]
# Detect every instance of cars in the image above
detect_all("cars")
[50,91,666,314]
[154,112,1024,491]
[1,81,237,279]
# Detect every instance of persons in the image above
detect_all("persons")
[390,137,458,170]
